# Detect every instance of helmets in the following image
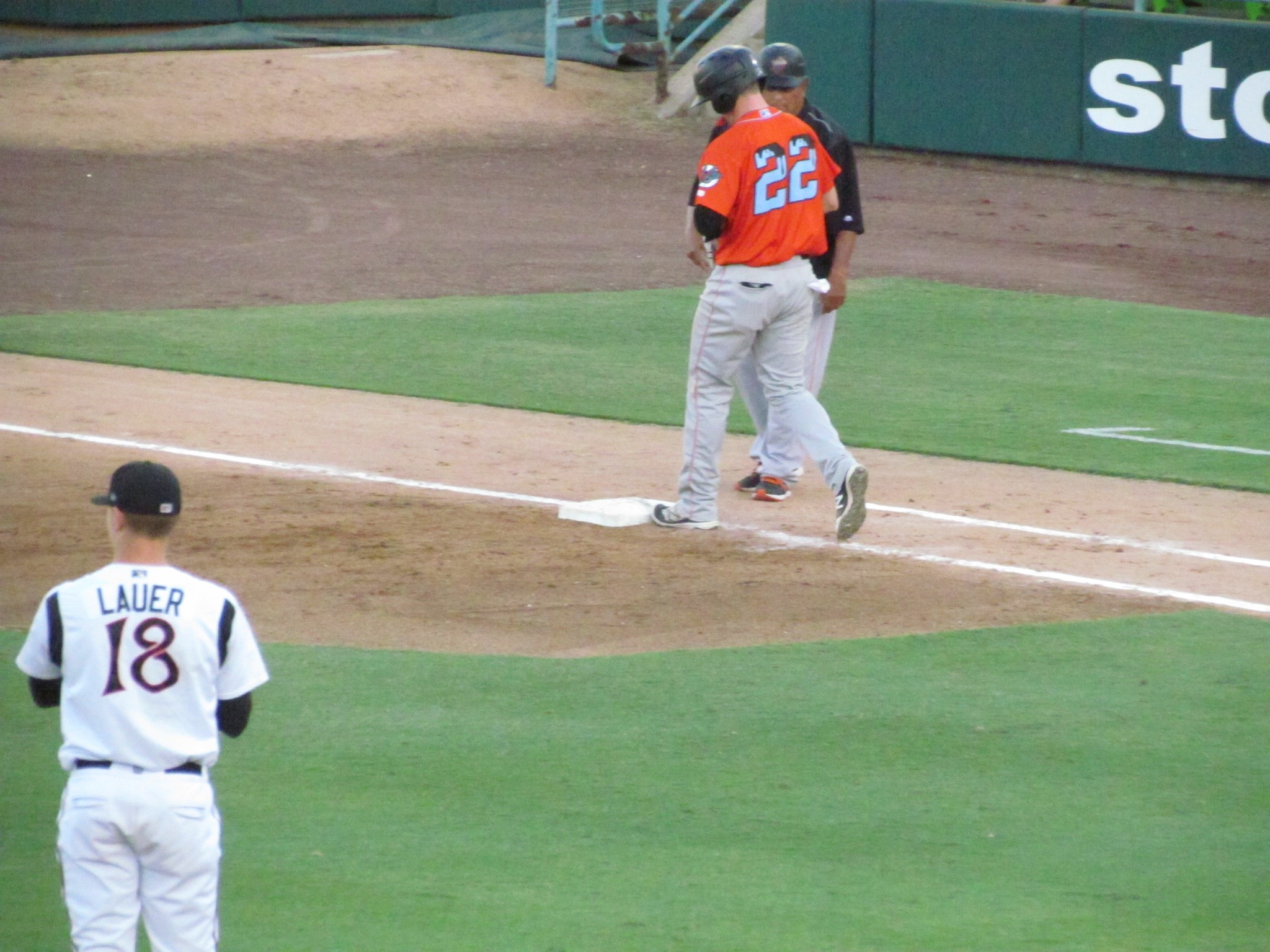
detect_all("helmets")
[759,43,805,87]
[690,45,767,114]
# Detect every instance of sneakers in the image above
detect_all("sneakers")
[735,462,761,491]
[651,504,718,530]
[834,464,868,539]
[752,476,792,501]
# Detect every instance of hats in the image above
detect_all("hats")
[91,461,181,515]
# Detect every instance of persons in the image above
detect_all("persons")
[682,42,865,502]
[651,46,868,542]
[13,462,269,952]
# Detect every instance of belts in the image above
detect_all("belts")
[76,759,201,775]
